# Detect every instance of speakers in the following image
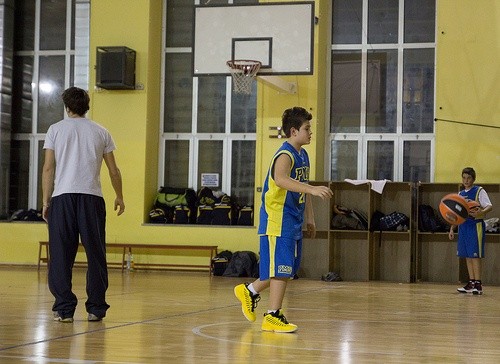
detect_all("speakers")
[101,52,125,82]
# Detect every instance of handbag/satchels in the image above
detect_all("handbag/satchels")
[385,212,410,231]
[195,186,234,225]
[15,209,45,221]
[150,186,198,224]
[222,251,258,277]
[211,250,235,276]
[484,217,500,234]
[331,205,368,230]
[370,210,387,231]
[232,204,254,226]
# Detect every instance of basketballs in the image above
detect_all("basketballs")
[466,200,481,218]
[437,193,468,227]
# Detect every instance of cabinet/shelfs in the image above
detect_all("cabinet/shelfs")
[299,179,416,282]
[415,180,500,287]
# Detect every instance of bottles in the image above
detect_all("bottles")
[127,252,135,272]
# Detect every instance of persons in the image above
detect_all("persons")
[235,105,335,334]
[448,167,492,294]
[42,87,125,322]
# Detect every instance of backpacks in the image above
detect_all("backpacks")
[418,204,437,233]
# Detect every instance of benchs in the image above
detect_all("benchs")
[37,240,218,276]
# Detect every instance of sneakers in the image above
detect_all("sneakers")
[472,281,483,295]
[233,282,261,322]
[87,313,101,321]
[53,310,73,322]
[261,307,297,332]
[457,281,474,293]
